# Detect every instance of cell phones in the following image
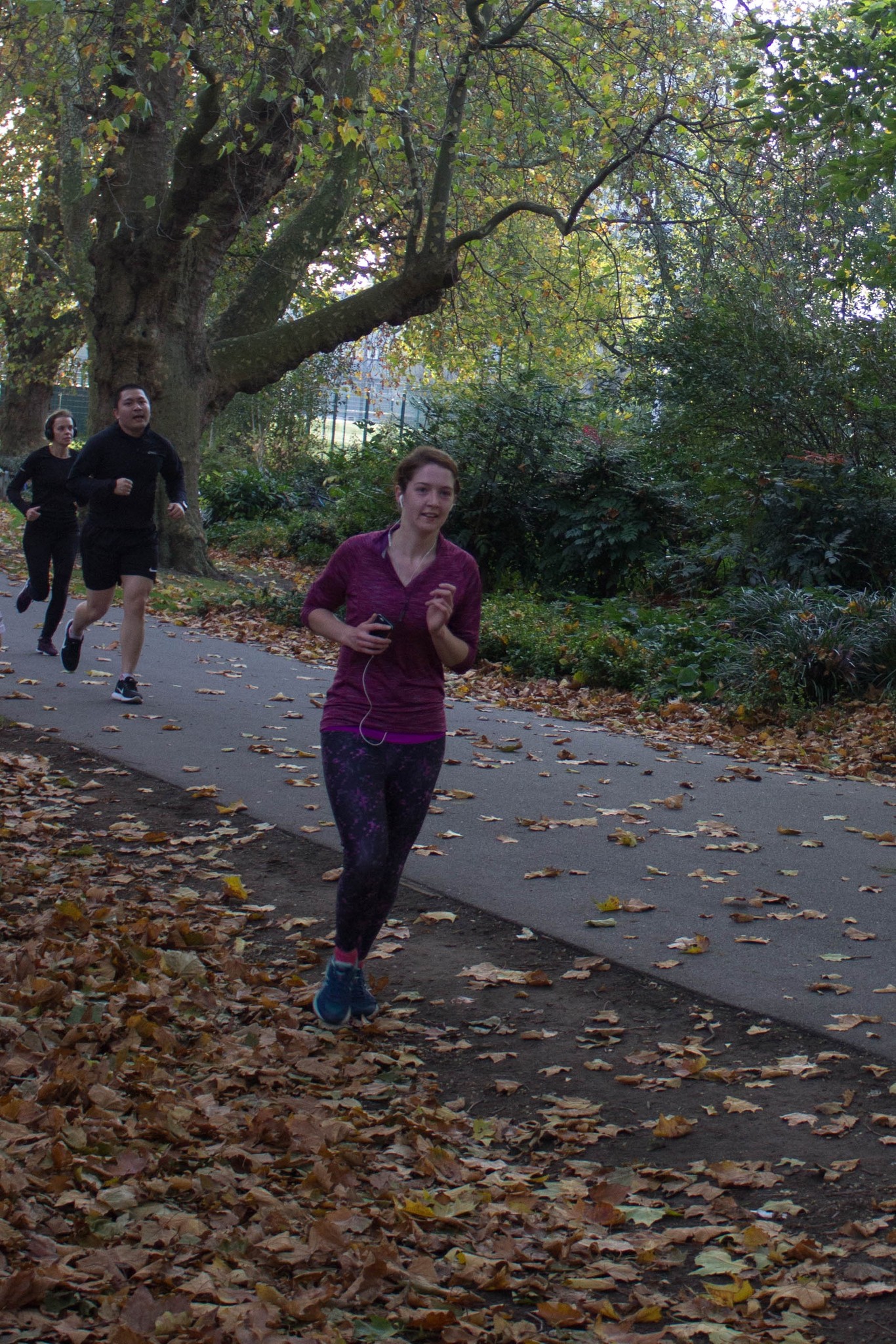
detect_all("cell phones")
[369,614,393,651]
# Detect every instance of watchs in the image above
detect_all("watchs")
[176,500,188,512]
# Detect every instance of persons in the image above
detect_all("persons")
[6,408,91,656]
[300,445,482,1027]
[59,384,188,699]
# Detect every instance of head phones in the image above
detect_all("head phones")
[45,414,77,440]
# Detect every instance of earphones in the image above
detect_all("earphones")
[450,504,453,511]
[399,494,403,507]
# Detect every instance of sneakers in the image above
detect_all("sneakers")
[60,619,84,673]
[36,638,58,656]
[16,578,32,613]
[350,969,380,1020]
[111,676,144,704]
[313,956,356,1027]
